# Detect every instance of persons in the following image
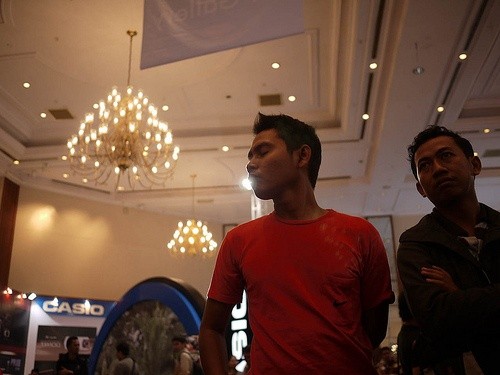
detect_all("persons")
[110,342,139,375]
[55,336,88,375]
[376,346,402,375]
[198,111,395,375]
[395,124,500,375]
[164,335,251,375]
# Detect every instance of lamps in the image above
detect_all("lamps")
[166,173,218,262]
[54,30,181,195]
